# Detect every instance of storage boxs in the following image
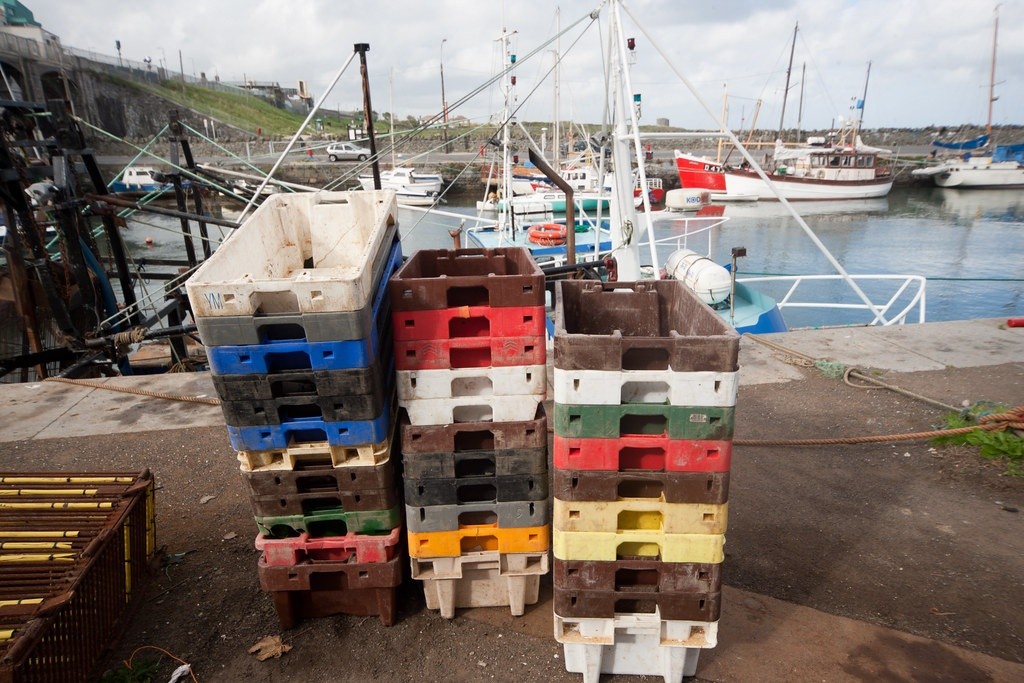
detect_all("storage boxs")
[185,190,742,683]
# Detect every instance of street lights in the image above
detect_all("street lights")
[440,38,450,153]
[731,247,746,325]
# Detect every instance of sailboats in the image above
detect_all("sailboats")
[673,20,895,202]
[357,73,447,206]
[310,0,928,334]
[477,2,665,213]
[911,2,1024,189]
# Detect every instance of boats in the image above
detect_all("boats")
[112,167,191,192]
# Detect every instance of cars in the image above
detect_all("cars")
[559,140,612,158]
[326,142,372,161]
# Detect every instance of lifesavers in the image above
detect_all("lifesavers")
[530,225,567,246]
